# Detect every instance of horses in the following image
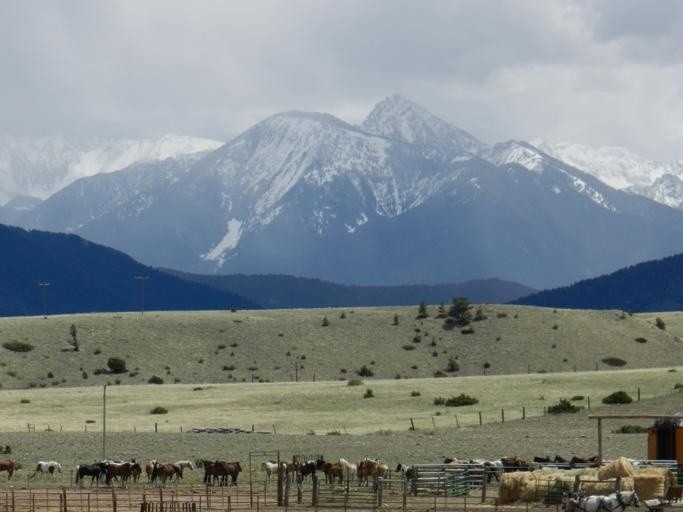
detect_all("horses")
[259,457,389,484]
[0,459,16,481]
[395,463,420,494]
[444,452,604,485]
[74,459,243,485]
[583,488,641,512]
[29,460,63,479]
